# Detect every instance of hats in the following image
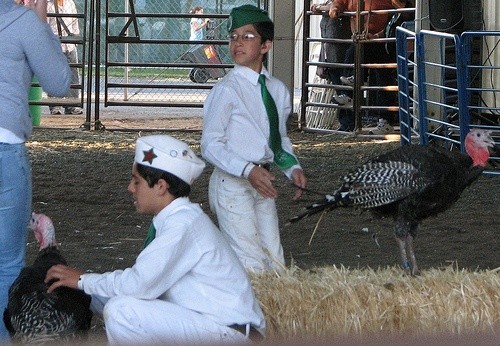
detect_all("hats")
[135,135,206,186]
[227,4,272,32]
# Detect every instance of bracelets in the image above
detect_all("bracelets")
[374,33,379,38]
[243,162,255,180]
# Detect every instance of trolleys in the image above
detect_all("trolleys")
[180,21,227,83]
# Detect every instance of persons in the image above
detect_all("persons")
[189,6,210,47]
[200,5,308,277]
[0,0,83,345]
[310,0,407,134]
[44,134,268,346]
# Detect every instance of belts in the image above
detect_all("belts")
[228,323,264,341]
[254,163,271,170]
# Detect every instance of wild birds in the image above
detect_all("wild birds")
[279,128,495,278]
[3,211,92,346]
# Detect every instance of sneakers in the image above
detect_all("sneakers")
[340,75,354,85]
[369,119,394,135]
[333,94,353,105]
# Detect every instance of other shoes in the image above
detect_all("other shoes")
[337,125,355,132]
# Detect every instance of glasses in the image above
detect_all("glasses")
[226,32,267,41]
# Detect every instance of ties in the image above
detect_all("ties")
[144,222,156,248]
[258,74,297,169]
